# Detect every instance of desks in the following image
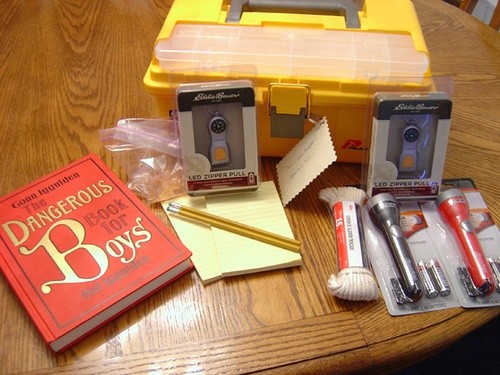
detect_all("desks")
[0,0,500,375]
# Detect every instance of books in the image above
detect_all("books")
[1,153,195,355]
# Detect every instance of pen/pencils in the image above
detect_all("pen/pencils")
[168,201,302,253]
